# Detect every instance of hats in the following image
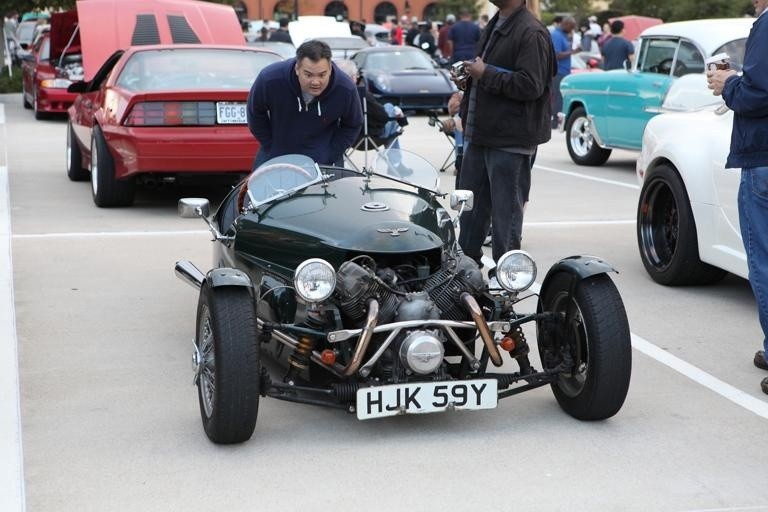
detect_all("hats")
[400,13,457,28]
[553,15,597,29]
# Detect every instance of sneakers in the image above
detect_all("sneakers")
[753,350,768,395]
[386,164,414,179]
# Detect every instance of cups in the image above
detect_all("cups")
[704,52,730,73]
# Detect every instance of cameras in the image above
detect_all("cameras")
[453,61,465,77]
[705,54,730,71]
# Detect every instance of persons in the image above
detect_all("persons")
[334,57,417,180]
[705,1,768,394]
[240,15,291,44]
[382,13,491,72]
[247,41,364,185]
[455,1,556,303]
[547,13,634,129]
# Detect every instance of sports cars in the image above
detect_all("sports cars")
[174,147,633,445]
